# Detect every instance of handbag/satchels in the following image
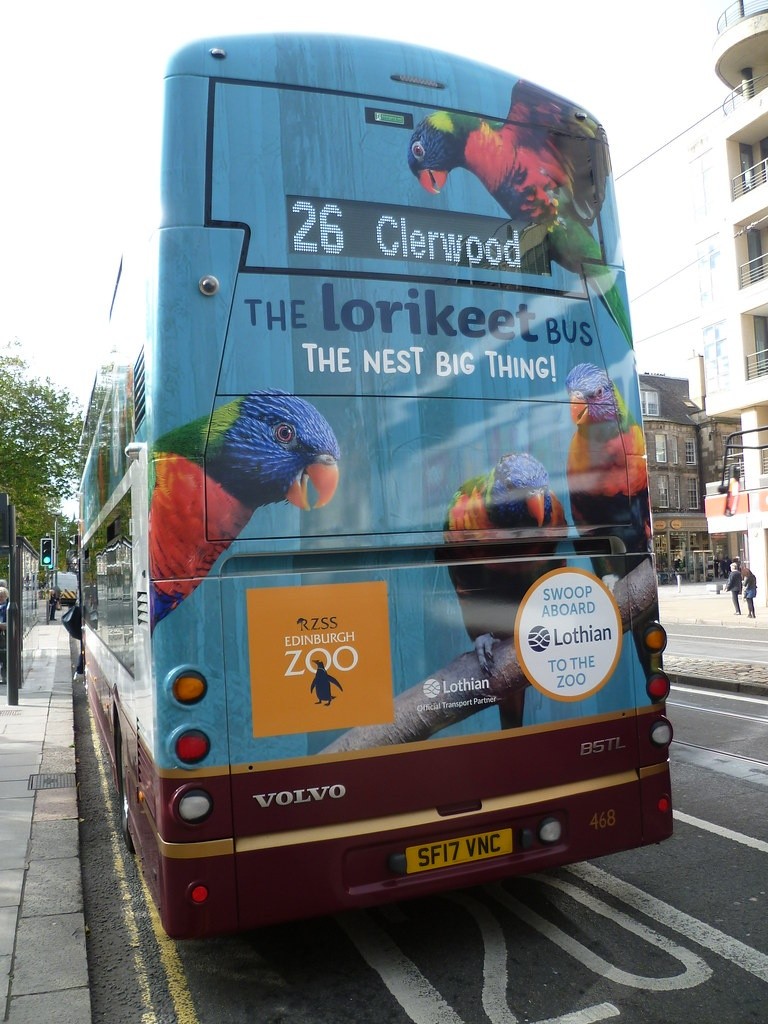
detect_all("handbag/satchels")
[60,606,83,640]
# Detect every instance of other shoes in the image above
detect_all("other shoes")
[735,612,741,615]
[0,680,7,684]
[74,671,84,681]
[748,614,752,618]
[51,619,57,621]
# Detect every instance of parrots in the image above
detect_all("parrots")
[408,80,634,351]
[150,387,343,636]
[441,451,567,730]
[566,360,659,704]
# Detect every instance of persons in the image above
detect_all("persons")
[0,579,9,685]
[713,556,758,619]
[71,596,83,680]
[673,557,685,569]
[49,589,59,624]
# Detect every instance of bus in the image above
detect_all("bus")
[73,31,680,944]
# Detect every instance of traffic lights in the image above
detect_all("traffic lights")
[38,538,54,568]
